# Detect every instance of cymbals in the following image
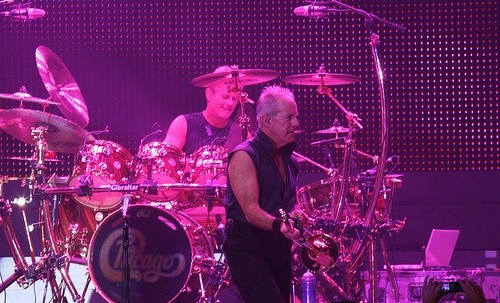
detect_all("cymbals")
[0,108,97,154]
[295,129,307,134]
[0,91,61,106]
[314,125,359,134]
[35,45,90,127]
[282,72,361,85]
[191,66,279,87]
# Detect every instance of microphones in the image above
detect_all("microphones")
[370,154,396,170]
[292,6,329,17]
[3,9,44,18]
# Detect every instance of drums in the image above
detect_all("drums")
[297,175,359,229]
[358,165,397,238]
[51,192,108,266]
[66,139,135,210]
[137,140,185,202]
[187,145,234,203]
[88,203,214,303]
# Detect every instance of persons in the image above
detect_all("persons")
[162,66,253,184]
[222,86,310,303]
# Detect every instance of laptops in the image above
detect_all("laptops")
[384,229,460,270]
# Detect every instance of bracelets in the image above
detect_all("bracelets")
[272,218,283,233]
